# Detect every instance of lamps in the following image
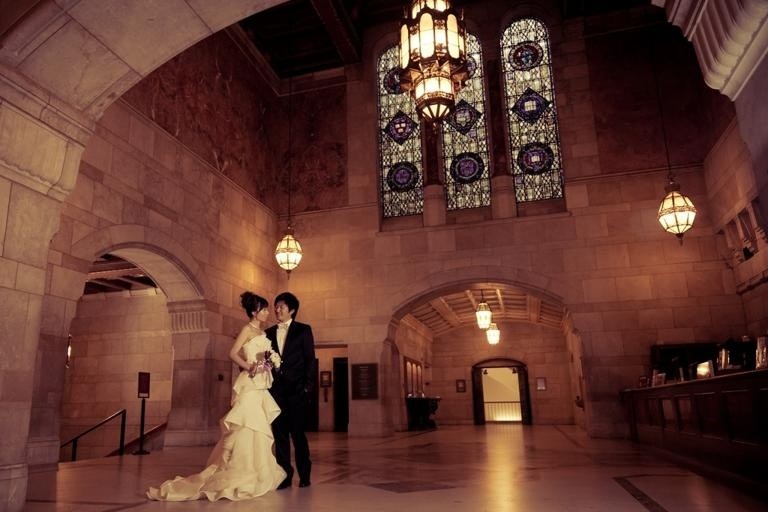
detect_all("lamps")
[394,0,470,134]
[275,74,303,279]
[486,323,501,346]
[651,59,698,247]
[475,289,492,330]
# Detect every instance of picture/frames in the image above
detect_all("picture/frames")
[320,371,331,389]
[536,377,547,390]
[457,379,466,393]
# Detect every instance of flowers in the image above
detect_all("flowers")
[251,351,282,377]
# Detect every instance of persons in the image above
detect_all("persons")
[261,292,319,489]
[146,292,288,502]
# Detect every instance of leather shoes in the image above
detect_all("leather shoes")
[299,481,310,487]
[278,481,291,489]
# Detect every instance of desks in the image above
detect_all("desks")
[408,397,441,430]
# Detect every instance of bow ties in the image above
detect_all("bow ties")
[278,323,288,329]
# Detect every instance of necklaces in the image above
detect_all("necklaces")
[248,322,261,331]
